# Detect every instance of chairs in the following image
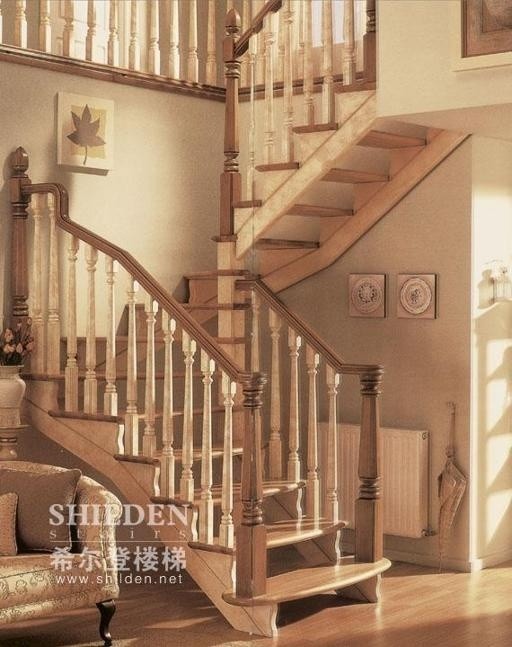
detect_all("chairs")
[0,459,123,647]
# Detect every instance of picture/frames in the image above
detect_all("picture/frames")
[447,0,512,72]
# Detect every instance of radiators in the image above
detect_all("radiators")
[311,419,430,538]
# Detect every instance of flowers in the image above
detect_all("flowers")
[0,316,36,367]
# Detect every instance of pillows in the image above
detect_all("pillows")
[0,466,80,554]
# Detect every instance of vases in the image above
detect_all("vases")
[0,365,24,426]
